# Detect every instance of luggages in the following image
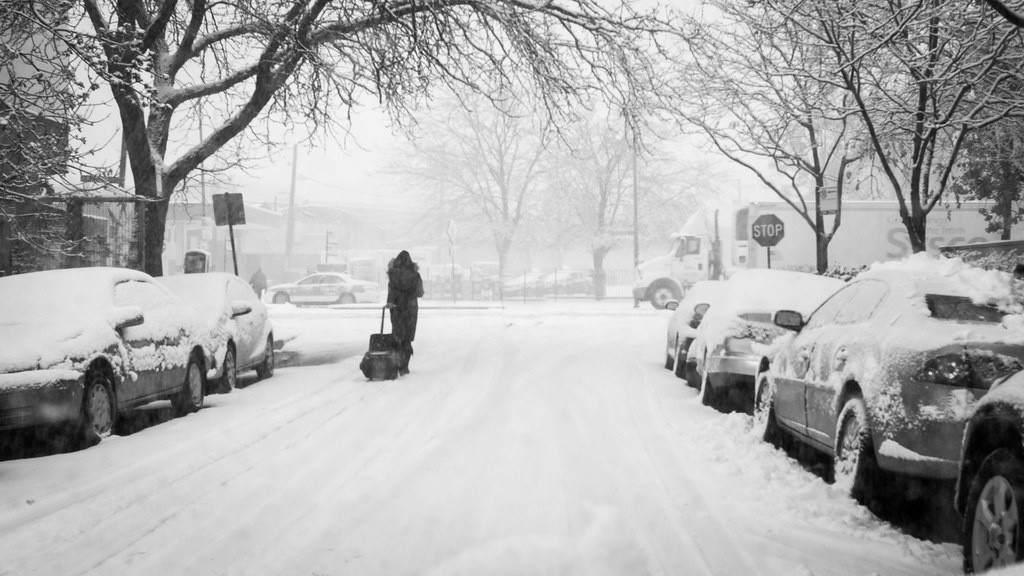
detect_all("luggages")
[360,306,401,380]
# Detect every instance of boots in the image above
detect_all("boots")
[400,347,413,376]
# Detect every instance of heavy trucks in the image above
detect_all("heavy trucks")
[632,201,1024,310]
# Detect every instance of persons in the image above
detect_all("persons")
[248,268,268,300]
[387,250,424,376]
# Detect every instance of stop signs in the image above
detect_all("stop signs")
[752,214,784,246]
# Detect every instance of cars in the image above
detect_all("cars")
[154,273,274,395]
[664,280,725,378]
[753,264,1024,576]
[424,268,591,297]
[686,269,847,411]
[0,266,213,450]
[264,272,381,304]
